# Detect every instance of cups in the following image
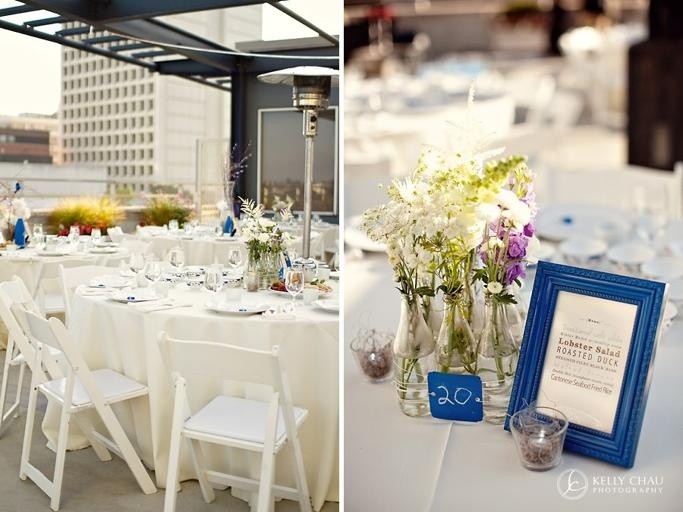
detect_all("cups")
[349,329,395,385]
[512,407,569,470]
[559,237,607,268]
[609,244,651,280]
[302,288,319,304]
[243,270,258,291]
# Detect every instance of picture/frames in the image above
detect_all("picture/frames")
[504,260,669,470]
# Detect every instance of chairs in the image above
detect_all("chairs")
[314,228,338,262]
[9,301,158,512]
[163,330,315,512]
[0,275,84,439]
[58,264,117,325]
[31,257,96,300]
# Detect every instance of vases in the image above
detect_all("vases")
[248,253,258,292]
[391,293,440,418]
[476,299,519,425]
[270,256,280,286]
[436,295,477,375]
[219,181,234,229]
[259,254,271,290]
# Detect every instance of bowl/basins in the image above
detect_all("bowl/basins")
[295,263,316,282]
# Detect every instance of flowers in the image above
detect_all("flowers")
[214,138,254,181]
[272,196,294,254]
[414,148,481,299]
[360,171,438,299]
[471,156,539,303]
[259,225,274,256]
[238,195,264,259]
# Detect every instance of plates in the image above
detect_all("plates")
[90,248,115,253]
[310,299,339,313]
[209,302,275,314]
[96,242,118,247]
[35,250,68,256]
[267,284,334,299]
[111,290,159,300]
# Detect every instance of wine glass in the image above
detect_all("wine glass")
[145,261,161,293]
[230,249,241,271]
[170,248,183,270]
[70,225,80,250]
[204,270,223,298]
[32,223,43,244]
[129,253,143,286]
[91,228,100,249]
[285,270,305,312]
